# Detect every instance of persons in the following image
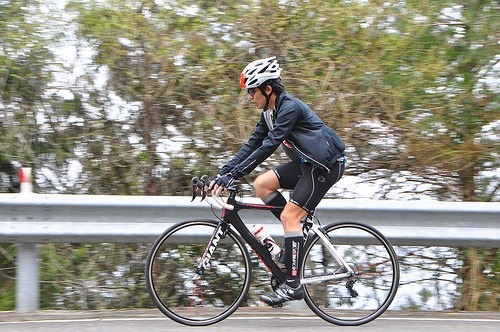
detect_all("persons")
[195,57,347,305]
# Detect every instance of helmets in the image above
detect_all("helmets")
[239,56,284,89]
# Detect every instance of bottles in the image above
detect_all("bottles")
[252,224,281,255]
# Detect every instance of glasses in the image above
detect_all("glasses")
[248,88,258,95]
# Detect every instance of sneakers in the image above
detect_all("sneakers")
[260,283,305,305]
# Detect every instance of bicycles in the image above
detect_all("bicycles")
[144,174,400,327]
